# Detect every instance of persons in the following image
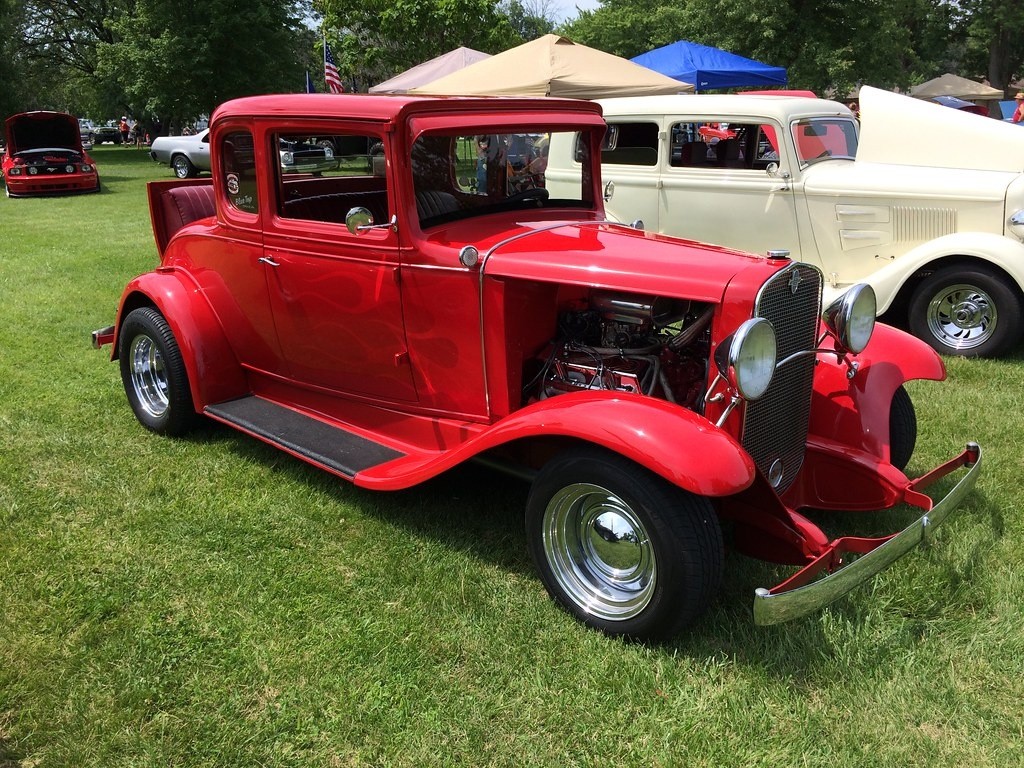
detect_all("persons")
[474,135,549,196]
[183,125,199,136]
[1012,92,1024,124]
[849,102,856,113]
[707,137,743,158]
[118,116,143,150]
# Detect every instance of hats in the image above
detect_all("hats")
[1014,93,1024,99]
[121,116,127,121]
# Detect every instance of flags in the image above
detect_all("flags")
[305,75,315,93]
[325,41,344,94]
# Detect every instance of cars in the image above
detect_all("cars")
[544,86,1024,358]
[90,90,982,637]
[368,129,545,178]
[700,91,849,159]
[151,123,340,179]
[76,117,135,144]
[1,110,102,194]
[81,134,92,150]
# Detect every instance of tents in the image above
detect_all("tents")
[629,40,785,141]
[407,34,695,102]
[911,73,1004,120]
[369,47,494,95]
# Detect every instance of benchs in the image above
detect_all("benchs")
[283,190,387,223]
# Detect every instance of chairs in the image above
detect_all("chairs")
[716,139,745,168]
[681,141,708,166]
[415,170,461,220]
